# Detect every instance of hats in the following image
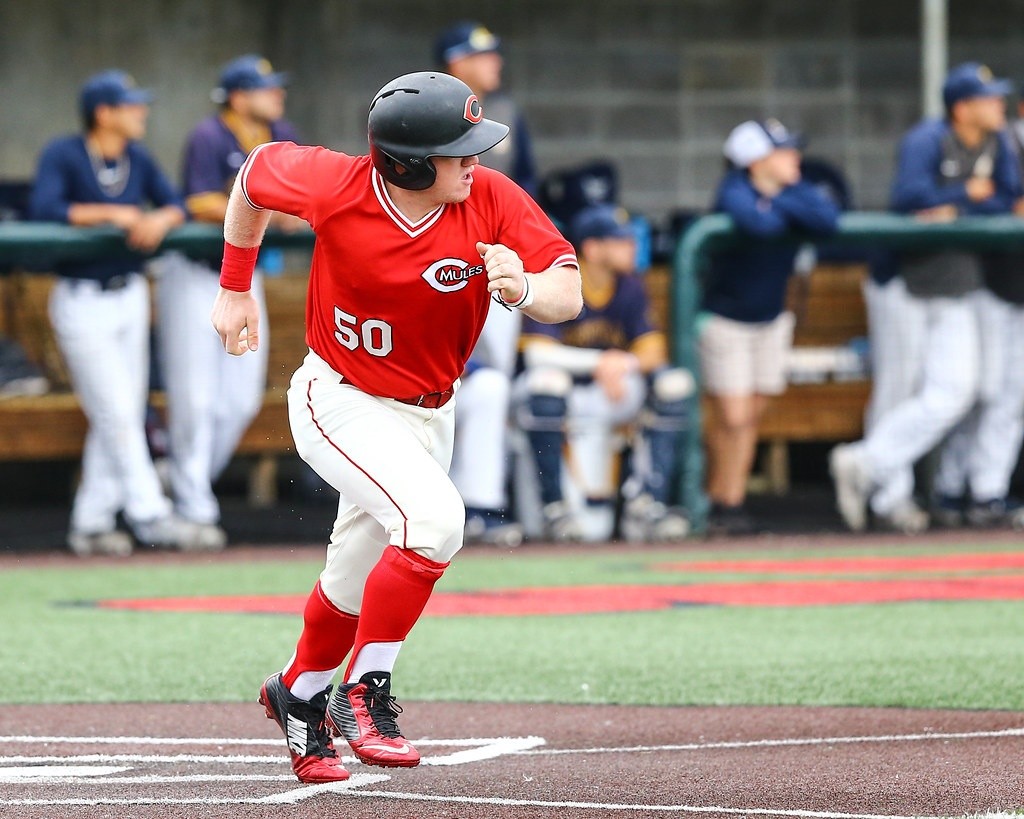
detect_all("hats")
[571,203,638,244]
[218,52,295,89]
[942,62,1015,107]
[436,18,502,65]
[80,68,155,108]
[721,116,811,170]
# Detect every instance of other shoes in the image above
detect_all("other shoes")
[827,439,878,531]
[470,515,522,549]
[134,515,231,555]
[926,484,1008,527]
[64,528,132,560]
[868,487,933,535]
[542,500,592,543]
[618,489,693,542]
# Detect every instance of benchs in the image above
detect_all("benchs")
[0,265,878,463]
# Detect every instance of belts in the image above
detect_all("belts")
[342,373,455,410]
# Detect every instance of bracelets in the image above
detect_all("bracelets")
[492,275,529,311]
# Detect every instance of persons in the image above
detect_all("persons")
[691,117,837,535]
[433,20,688,547]
[212,71,582,781]
[35,68,200,553]
[157,55,307,550]
[829,63,1024,532]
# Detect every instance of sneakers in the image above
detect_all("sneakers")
[327,671,420,769]
[257,670,350,782]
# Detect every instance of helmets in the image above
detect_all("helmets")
[366,70,510,191]
